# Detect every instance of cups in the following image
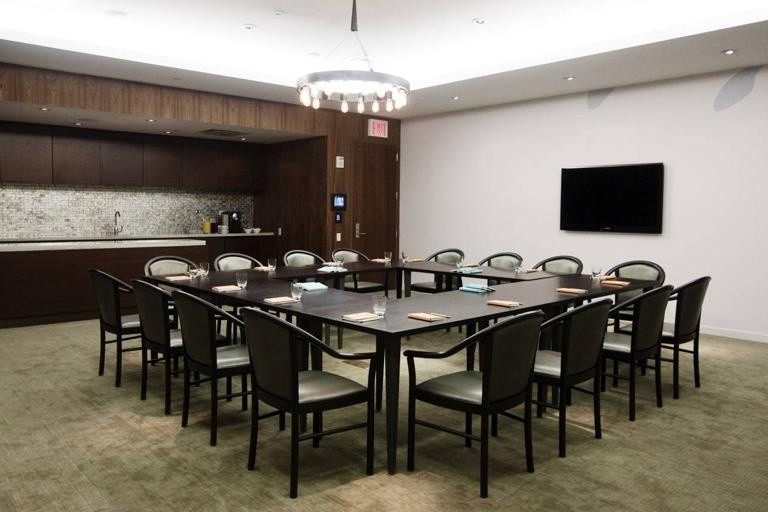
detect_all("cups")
[189,264,199,278]
[592,264,601,281]
[203,216,210,234]
[290,282,302,300]
[236,272,247,289]
[268,258,276,271]
[200,262,209,276]
[373,294,386,318]
[384,251,392,264]
[337,256,344,265]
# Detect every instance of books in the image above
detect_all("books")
[212,285,241,292]
[293,281,328,291]
[165,275,189,281]
[322,262,336,266]
[410,256,425,262]
[318,266,347,273]
[371,259,385,264]
[601,275,616,279]
[254,266,269,272]
[600,280,631,287]
[459,285,496,293]
[466,262,480,267]
[408,312,445,322]
[342,311,380,323]
[263,296,300,305]
[527,268,537,272]
[556,287,588,295]
[487,299,521,308]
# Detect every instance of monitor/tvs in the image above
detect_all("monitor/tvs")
[331,193,347,210]
[559,163,664,234]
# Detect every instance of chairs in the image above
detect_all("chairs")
[238,307,381,499]
[130,278,232,416]
[611,276,710,400]
[566,285,673,422]
[458,252,522,334]
[87,268,179,388]
[282,250,326,323]
[330,247,388,299]
[402,310,546,499]
[587,261,664,368]
[213,253,279,343]
[409,249,464,332]
[170,289,285,447]
[532,256,582,313]
[144,255,198,362]
[490,298,613,458]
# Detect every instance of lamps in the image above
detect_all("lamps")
[296,0,409,113]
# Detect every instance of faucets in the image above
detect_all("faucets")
[114,211,123,236]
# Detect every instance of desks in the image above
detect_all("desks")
[133,258,660,476]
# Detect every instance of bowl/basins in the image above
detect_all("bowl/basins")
[244,228,252,233]
[253,228,261,233]
